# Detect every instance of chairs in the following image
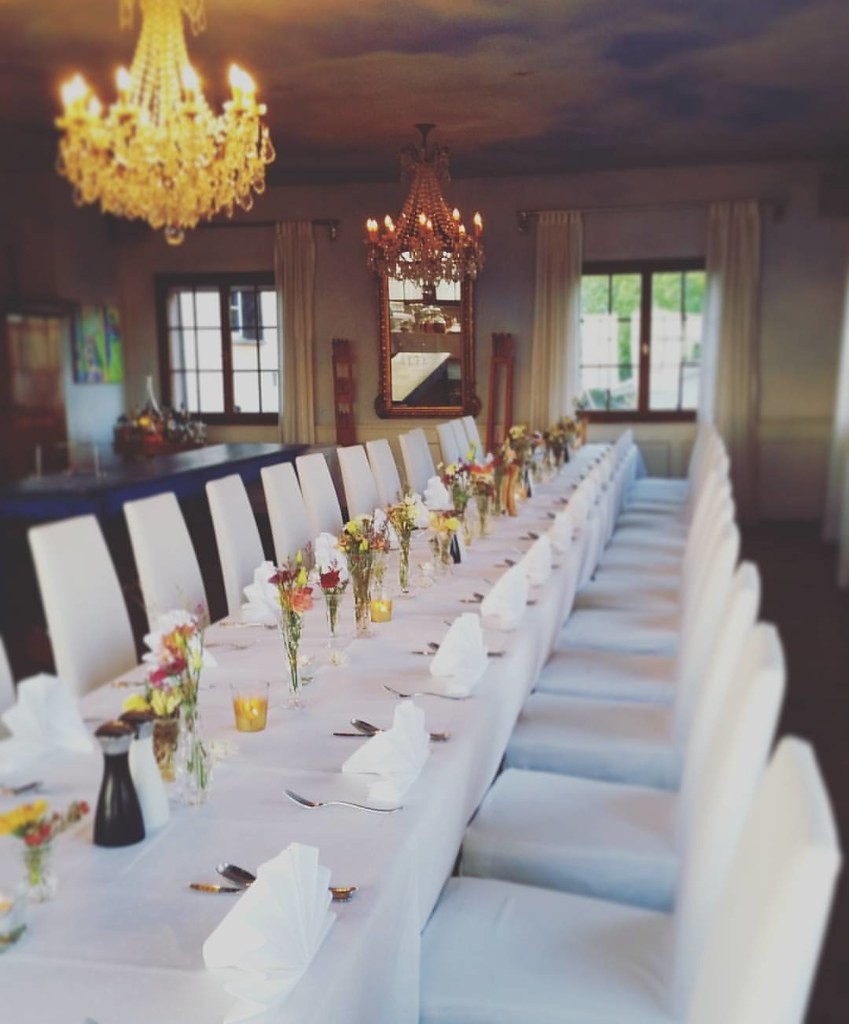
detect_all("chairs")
[397,432,427,493]
[121,490,213,635]
[551,415,738,648]
[531,519,742,698]
[295,452,344,538]
[260,461,314,571]
[413,737,841,1024]
[451,619,789,916]
[501,559,765,792]
[435,423,461,470]
[365,438,403,508]
[336,445,381,520]
[459,415,482,456]
[447,418,471,458]
[24,512,138,698]
[409,426,437,483]
[203,473,267,615]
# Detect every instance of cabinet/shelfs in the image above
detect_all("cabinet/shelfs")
[0,295,72,485]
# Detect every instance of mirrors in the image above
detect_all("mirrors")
[373,234,482,419]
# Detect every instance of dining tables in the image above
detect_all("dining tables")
[0,435,641,1024]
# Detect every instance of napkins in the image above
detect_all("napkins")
[312,532,348,583]
[480,562,527,628]
[200,840,337,1022]
[339,697,434,807]
[428,612,488,695]
[139,608,219,689]
[357,507,401,553]
[519,428,633,587]
[0,672,96,792]
[421,473,454,510]
[238,561,285,628]
[403,492,433,528]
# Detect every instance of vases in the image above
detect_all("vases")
[430,418,585,573]
[168,688,211,806]
[397,537,417,600]
[19,841,57,901]
[320,596,352,672]
[277,609,314,714]
[346,555,375,638]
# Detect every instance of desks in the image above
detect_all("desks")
[0,440,311,553]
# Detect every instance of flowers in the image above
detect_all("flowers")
[0,799,90,849]
[145,599,205,704]
[267,539,317,613]
[427,393,589,529]
[305,542,348,596]
[332,518,377,555]
[381,466,418,547]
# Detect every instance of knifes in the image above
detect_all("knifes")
[190,882,357,893]
[412,650,437,655]
[334,732,376,737]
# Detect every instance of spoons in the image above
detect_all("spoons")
[283,790,402,813]
[217,863,351,898]
[352,720,449,739]
[382,686,468,700]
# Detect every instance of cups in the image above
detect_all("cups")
[371,589,393,622]
[231,682,269,732]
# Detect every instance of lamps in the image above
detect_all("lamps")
[360,141,487,284]
[50,1,277,248]
